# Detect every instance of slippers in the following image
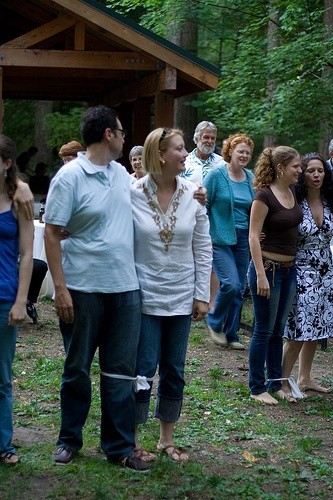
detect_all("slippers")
[158,445,192,463]
[134,447,155,463]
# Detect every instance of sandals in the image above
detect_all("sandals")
[0,452,21,467]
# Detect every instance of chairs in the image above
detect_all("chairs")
[15,258,48,325]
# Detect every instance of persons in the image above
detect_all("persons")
[280,152,333,399]
[129,145,148,178]
[325,138,333,170]
[43,107,150,472]
[201,134,257,349]
[249,146,304,405]
[59,139,85,164]
[0,134,48,465]
[58,127,213,461]
[179,121,227,308]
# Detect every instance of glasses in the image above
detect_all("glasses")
[112,127,125,138]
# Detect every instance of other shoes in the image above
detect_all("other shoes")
[238,363,250,370]
[230,342,245,349]
[209,326,227,345]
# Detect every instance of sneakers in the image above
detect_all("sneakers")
[120,455,150,472]
[53,444,73,463]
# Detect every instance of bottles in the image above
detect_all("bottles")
[38,202,45,223]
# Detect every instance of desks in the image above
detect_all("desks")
[32,219,56,302]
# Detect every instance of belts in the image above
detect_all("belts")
[279,262,294,267]
